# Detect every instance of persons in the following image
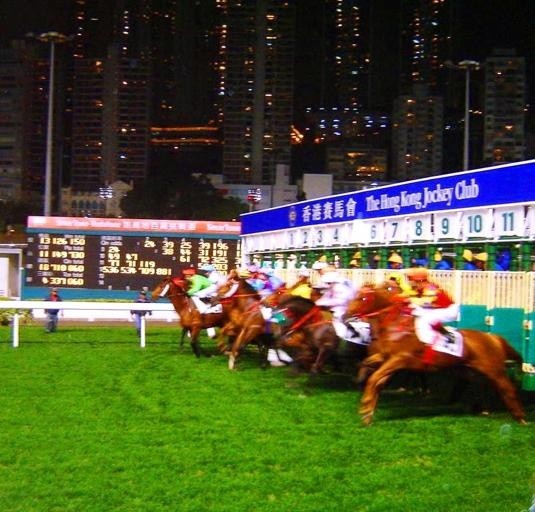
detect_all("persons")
[129,291,151,335]
[44,288,61,332]
[182,264,458,366]
[253,248,515,272]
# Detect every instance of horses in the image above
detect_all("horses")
[340,282,526,426]
[151,276,225,358]
[261,283,367,377]
[211,270,310,371]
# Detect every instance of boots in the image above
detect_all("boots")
[344,322,359,338]
[432,322,455,343]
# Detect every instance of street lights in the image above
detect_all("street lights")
[97,186,113,218]
[457,60,481,170]
[245,188,262,211]
[34,31,72,217]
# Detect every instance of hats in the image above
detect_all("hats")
[248,252,380,282]
[52,288,57,292]
[198,264,213,272]
[387,249,488,282]
[140,291,146,294]
[182,269,196,275]
